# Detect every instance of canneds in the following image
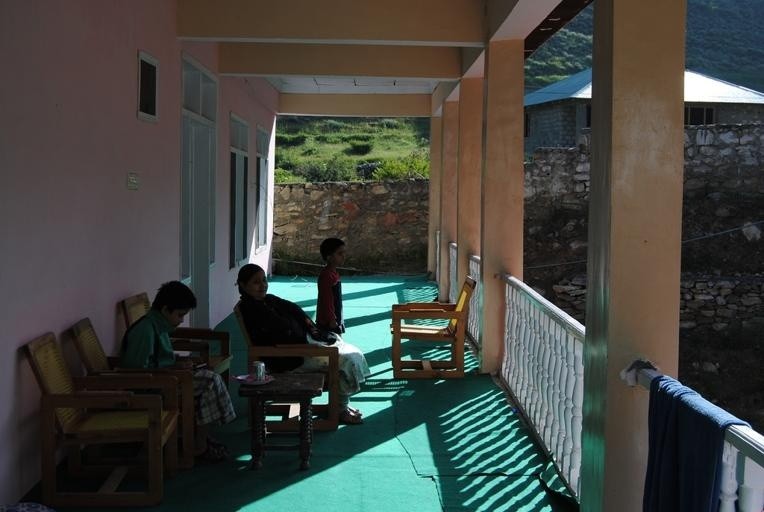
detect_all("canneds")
[249,361,266,381]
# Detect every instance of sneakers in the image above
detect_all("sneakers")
[339,407,362,424]
[192,435,229,467]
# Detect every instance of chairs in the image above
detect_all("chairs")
[236,307,340,436]
[118,290,234,394]
[21,330,181,510]
[389,277,477,379]
[67,316,201,470]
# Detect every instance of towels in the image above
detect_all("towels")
[642,372,752,512]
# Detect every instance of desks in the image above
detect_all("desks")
[240,374,325,473]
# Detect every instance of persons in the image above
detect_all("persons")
[316,238,345,337]
[118,281,237,464]
[239,264,371,425]
